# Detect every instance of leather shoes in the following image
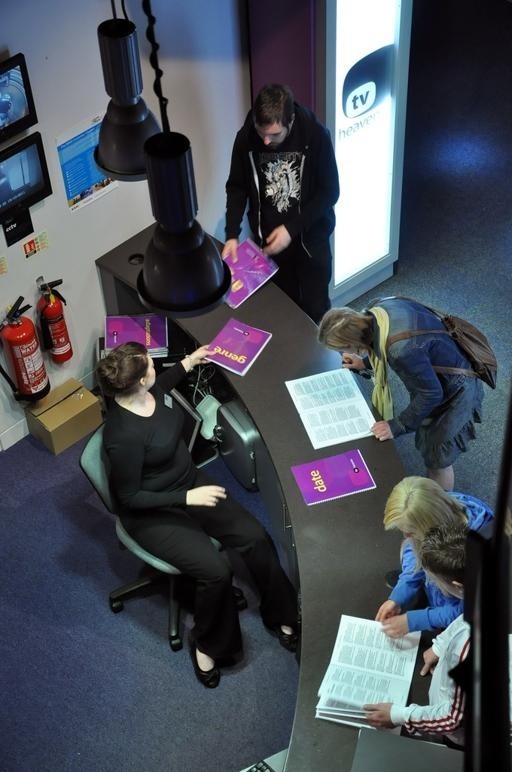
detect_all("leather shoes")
[188,631,220,688]
[276,625,298,651]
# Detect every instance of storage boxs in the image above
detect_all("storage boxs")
[24,378,104,456]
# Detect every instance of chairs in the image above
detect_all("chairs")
[79,424,221,651]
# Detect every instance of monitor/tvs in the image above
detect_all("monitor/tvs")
[0,130,53,227]
[0,53,38,141]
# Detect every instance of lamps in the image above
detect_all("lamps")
[94,0,162,181]
[134,0,231,319]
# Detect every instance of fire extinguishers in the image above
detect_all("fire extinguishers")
[36,279,73,364]
[0,296,51,402]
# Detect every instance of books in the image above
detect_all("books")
[221,237,280,310]
[290,449,377,507]
[203,318,273,376]
[105,315,170,358]
[315,613,415,730]
[284,368,376,450]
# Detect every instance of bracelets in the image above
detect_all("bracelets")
[184,354,195,372]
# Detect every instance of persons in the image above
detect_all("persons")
[317,296,485,493]
[95,341,300,690]
[220,82,340,325]
[373,475,497,640]
[363,523,473,751]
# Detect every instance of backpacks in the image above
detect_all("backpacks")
[368,297,498,389]
